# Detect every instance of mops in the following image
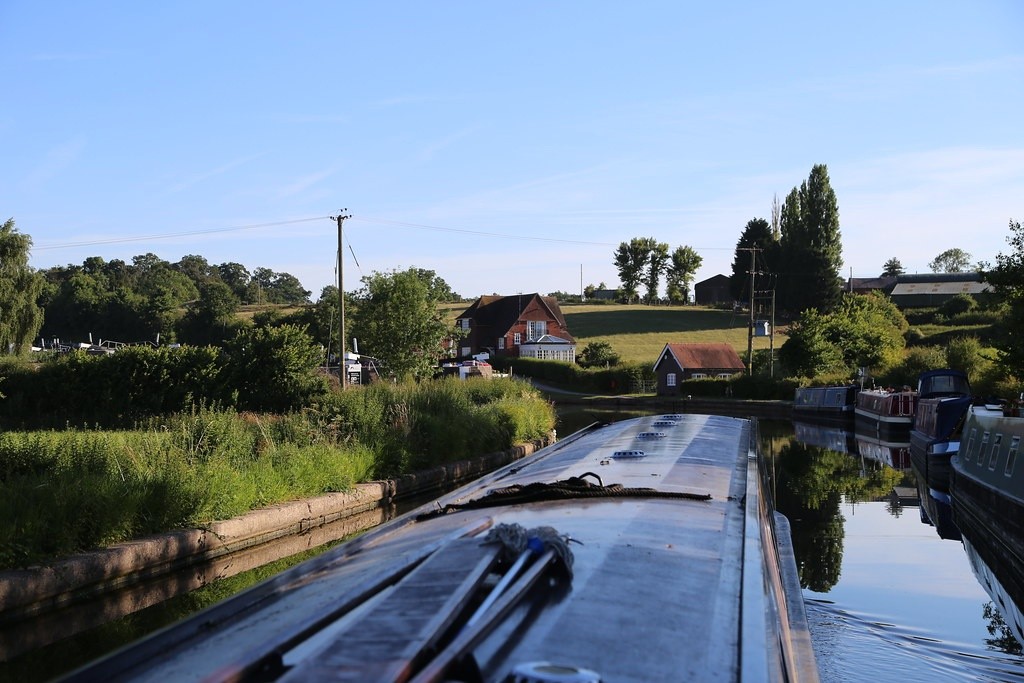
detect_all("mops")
[387,521,576,683]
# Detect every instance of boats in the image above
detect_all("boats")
[908,456,962,541]
[854,378,919,432]
[909,368,976,492]
[40,410,818,683]
[945,402,1024,566]
[948,518,1024,658]
[854,423,914,482]
[791,386,862,416]
